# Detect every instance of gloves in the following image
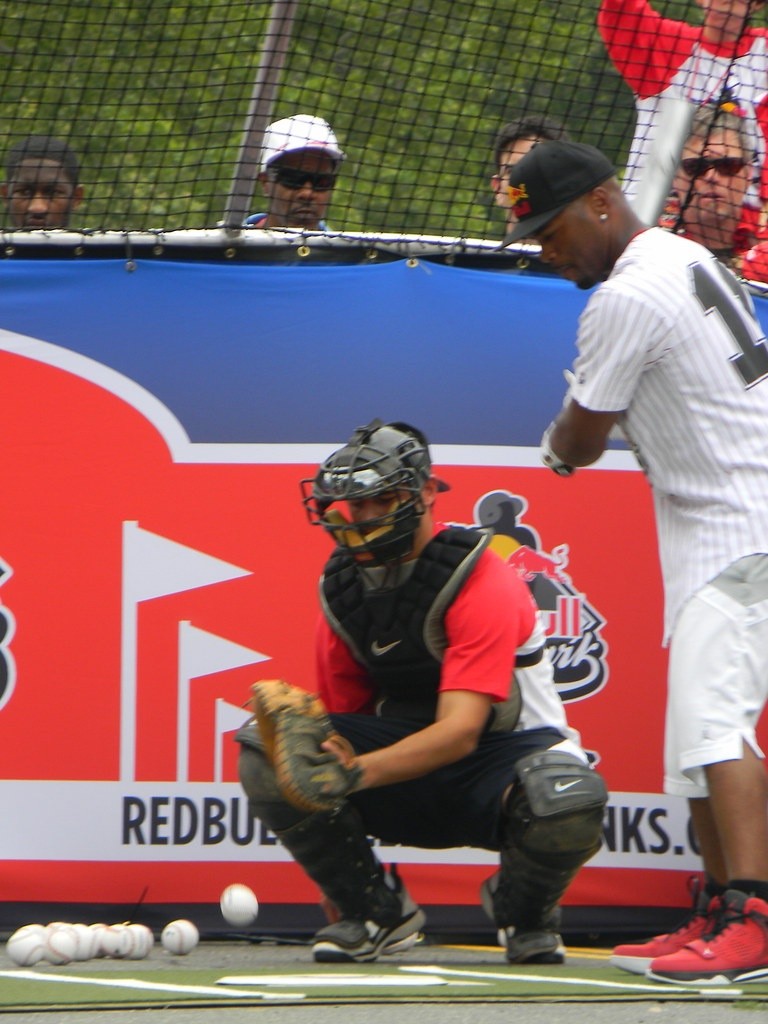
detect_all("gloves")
[541,368,576,477]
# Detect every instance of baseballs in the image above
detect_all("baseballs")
[219,883,258,927]
[127,924,155,959]
[161,919,199,956]
[90,923,130,961]
[5,923,46,966]
[71,923,96,962]
[43,922,78,965]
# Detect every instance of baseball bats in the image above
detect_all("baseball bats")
[550,96,695,479]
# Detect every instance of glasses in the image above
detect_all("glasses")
[264,165,339,192]
[496,166,512,179]
[680,153,759,180]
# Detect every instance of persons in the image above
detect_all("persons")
[600,0,768,219]
[492,116,575,244]
[659,104,768,283]
[493,141,768,985]
[0,140,85,230]
[234,419,609,966]
[246,115,347,232]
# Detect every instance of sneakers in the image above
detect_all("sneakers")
[312,862,425,962]
[480,872,565,965]
[610,877,726,974]
[647,888,768,984]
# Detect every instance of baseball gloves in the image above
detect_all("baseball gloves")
[248,680,366,812]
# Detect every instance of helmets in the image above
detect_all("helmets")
[300,419,452,597]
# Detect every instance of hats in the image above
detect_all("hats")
[494,138,618,253]
[258,115,349,174]
